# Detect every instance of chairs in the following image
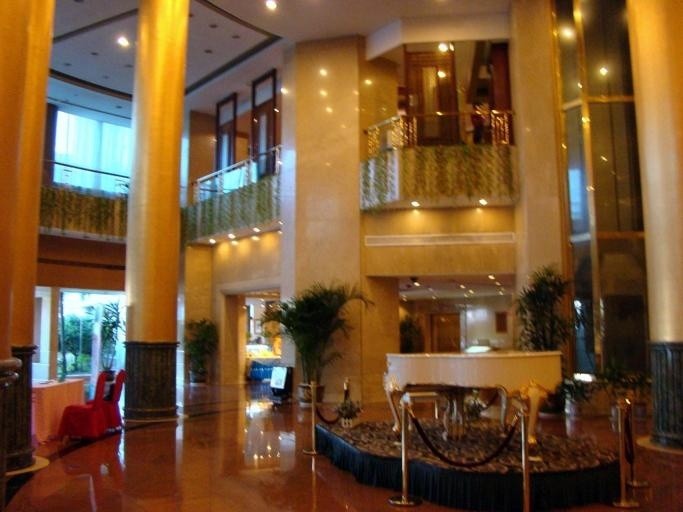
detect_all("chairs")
[59,371,110,442]
[86,369,127,432]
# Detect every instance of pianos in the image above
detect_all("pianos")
[383,351,562,444]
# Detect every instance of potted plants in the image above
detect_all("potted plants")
[259,284,375,408]
[182,319,216,389]
[509,261,573,416]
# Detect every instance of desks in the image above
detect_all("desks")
[29,379,84,442]
